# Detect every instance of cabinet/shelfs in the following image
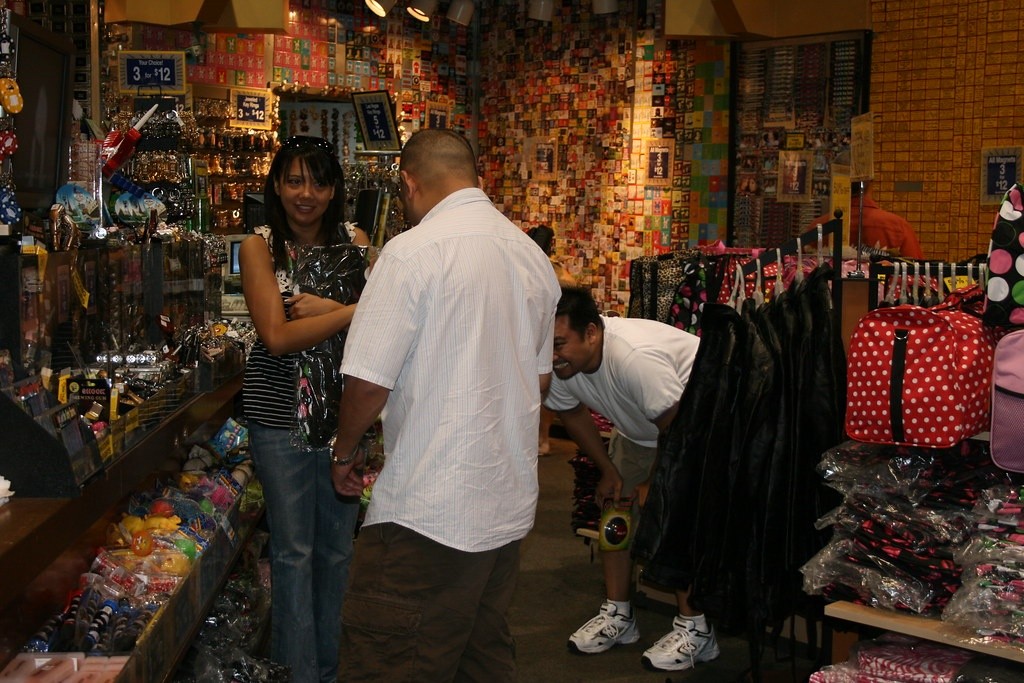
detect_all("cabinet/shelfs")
[823,424,1024,662]
[0,320,268,683]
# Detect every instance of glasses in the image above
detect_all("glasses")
[285,135,333,155]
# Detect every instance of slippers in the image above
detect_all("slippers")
[538,450,551,456]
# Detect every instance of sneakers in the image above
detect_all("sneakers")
[568,602,640,654]
[642,615,720,671]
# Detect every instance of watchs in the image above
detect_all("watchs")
[327,433,360,465]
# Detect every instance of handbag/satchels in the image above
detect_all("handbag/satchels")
[808,633,1024,683]
[669,238,834,337]
[844,181,1024,474]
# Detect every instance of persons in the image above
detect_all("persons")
[328,128,562,683]
[849,177,923,261]
[525,225,577,457]
[543,285,721,672]
[238,135,371,683]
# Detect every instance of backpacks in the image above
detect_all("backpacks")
[293,244,384,449]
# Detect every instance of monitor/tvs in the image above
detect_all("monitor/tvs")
[226,233,246,286]
